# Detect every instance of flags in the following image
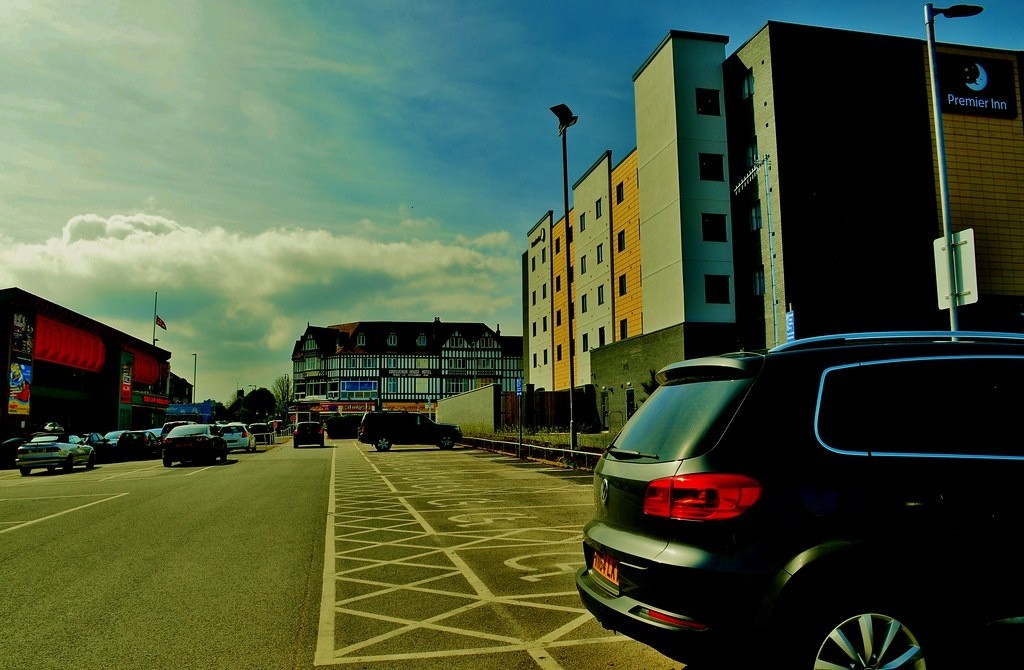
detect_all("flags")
[156,315,167,330]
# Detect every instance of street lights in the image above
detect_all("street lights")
[925,3,984,340]
[192,353,196,404]
[550,104,579,450]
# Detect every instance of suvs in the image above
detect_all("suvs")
[357,410,462,451]
[574,330,1024,670]
[293,422,325,448]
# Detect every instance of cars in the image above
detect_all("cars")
[68,433,115,464]
[0,432,40,470]
[220,424,257,452]
[104,428,164,459]
[162,424,227,466]
[268,420,287,436]
[248,423,272,443]
[16,431,97,476]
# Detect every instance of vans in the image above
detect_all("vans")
[162,421,197,448]
